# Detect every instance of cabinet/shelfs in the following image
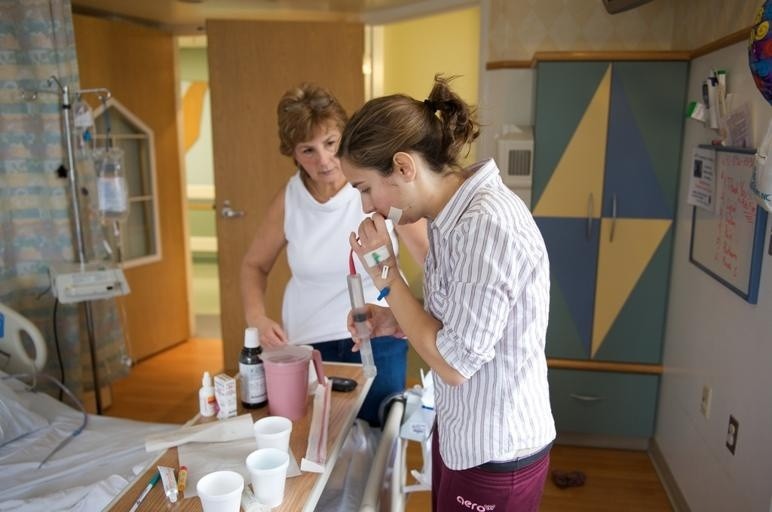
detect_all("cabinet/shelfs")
[530,50,685,452]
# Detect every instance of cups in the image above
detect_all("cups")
[245,447,291,509]
[257,344,327,423]
[195,469,245,512]
[252,415,293,455]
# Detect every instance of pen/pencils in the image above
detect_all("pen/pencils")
[128,471,160,512]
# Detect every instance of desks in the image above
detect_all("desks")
[103,360,378,512]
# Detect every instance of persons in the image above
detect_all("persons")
[334,71,556,511]
[238,78,412,494]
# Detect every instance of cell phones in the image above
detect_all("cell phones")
[330,376,357,392]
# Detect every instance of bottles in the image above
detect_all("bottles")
[237,328,267,410]
[198,371,217,416]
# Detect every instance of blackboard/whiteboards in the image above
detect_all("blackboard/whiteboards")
[689,143,767,304]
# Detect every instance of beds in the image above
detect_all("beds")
[1,303,410,512]
